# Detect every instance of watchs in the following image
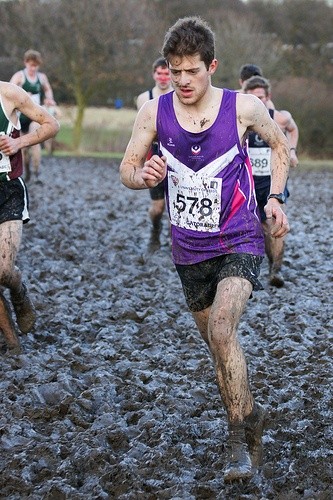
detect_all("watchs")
[267,193,286,204]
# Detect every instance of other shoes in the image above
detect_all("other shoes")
[245,402,265,467]
[148,224,163,251]
[221,443,252,480]
[10,282,35,331]
[270,273,283,287]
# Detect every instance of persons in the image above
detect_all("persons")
[10,51,57,187]
[137,57,176,254]
[119,17,291,483]
[232,65,299,289]
[0,75,61,350]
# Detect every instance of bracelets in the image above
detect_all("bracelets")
[290,147,296,152]
